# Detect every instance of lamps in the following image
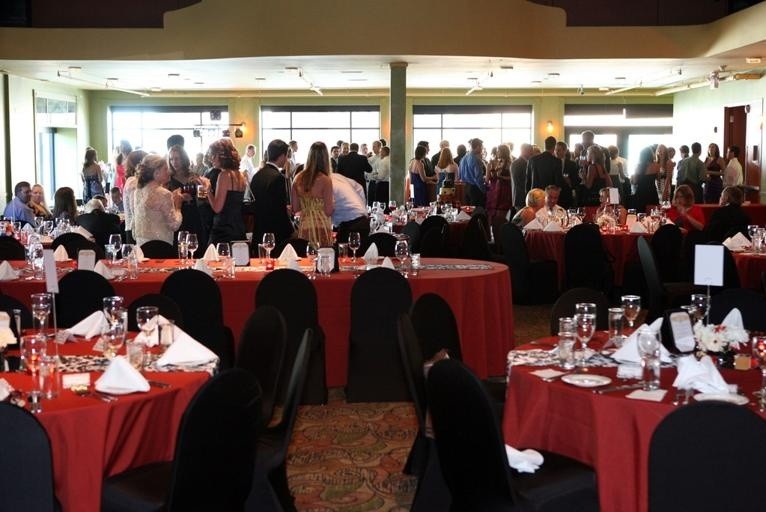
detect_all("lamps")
[238,123,247,135]
[546,120,555,133]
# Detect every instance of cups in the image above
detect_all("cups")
[606,306,622,338]
[756,228,766,250]
[691,293,713,321]
[641,358,661,389]
[555,331,577,368]
[157,319,175,347]
[36,358,59,398]
[558,318,577,339]
[125,337,143,370]
[196,185,207,198]
[577,302,598,340]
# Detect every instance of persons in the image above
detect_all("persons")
[248,139,298,258]
[291,140,369,267]
[26,184,52,218]
[679,185,752,267]
[113,139,132,196]
[75,198,124,250]
[630,143,743,214]
[123,151,183,259]
[410,139,516,226]
[535,184,566,218]
[239,144,256,173]
[666,185,704,236]
[510,188,547,230]
[111,187,123,212]
[80,146,108,203]
[92,195,108,207]
[511,131,630,210]
[163,135,247,258]
[3,182,41,228]
[364,139,389,206]
[35,187,78,236]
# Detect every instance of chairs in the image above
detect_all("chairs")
[647,401,765,511]
[7,404,61,510]
[255,268,329,405]
[242,328,312,511]
[409,357,600,510]
[344,268,421,402]
[410,291,507,416]
[0,177,766,376]
[395,313,432,474]
[103,360,269,510]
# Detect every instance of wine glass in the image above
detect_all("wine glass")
[101,294,128,354]
[30,293,53,336]
[21,336,45,375]
[604,207,666,232]
[751,335,766,395]
[622,294,641,332]
[576,316,595,373]
[535,207,586,230]
[634,324,663,392]
[135,306,158,349]
[748,225,759,252]
[0,217,77,281]
[365,200,465,225]
[183,184,193,203]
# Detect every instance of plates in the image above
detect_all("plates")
[692,392,748,407]
[560,374,612,387]
[161,358,216,368]
[98,383,147,396]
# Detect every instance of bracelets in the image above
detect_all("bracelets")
[176,207,181,209]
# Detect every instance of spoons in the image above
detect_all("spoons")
[673,388,692,405]
[71,384,116,405]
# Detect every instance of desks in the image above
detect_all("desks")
[7,328,218,511]
[503,329,765,509]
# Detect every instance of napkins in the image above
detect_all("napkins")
[612,308,750,394]
[134,316,221,370]
[95,355,151,396]
[66,310,111,341]
